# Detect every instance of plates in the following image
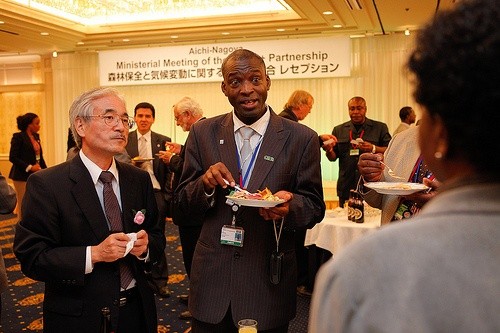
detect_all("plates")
[133,158,155,162]
[155,153,162,156]
[364,182,429,195]
[225,195,286,207]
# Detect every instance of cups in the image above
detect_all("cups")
[345,200,348,213]
[237,319,258,333]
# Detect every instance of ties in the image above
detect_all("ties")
[139,137,151,174]
[100,171,133,289]
[239,127,255,189]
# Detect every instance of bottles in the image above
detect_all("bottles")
[347,189,364,223]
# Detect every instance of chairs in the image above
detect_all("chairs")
[296,244,333,298]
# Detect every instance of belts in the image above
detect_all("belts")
[112,295,133,308]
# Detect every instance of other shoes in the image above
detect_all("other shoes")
[179,295,189,300]
[179,311,190,319]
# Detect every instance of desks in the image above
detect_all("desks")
[304,210,381,258]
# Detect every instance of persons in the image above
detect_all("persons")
[13,87,166,333]
[323,97,392,208]
[393,107,416,135]
[124,102,171,296]
[9,113,47,221]
[66,144,132,164]
[308,0,500,333]
[160,97,206,169]
[174,49,327,333]
[278,90,338,147]
[357,126,442,226]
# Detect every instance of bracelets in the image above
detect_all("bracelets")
[372,145,376,153]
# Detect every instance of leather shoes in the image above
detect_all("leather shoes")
[158,286,171,297]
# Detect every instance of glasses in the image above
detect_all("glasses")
[86,114,135,129]
[175,110,187,121]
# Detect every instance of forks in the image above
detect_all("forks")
[380,159,406,180]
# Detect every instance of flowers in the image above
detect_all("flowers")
[131,209,146,225]
